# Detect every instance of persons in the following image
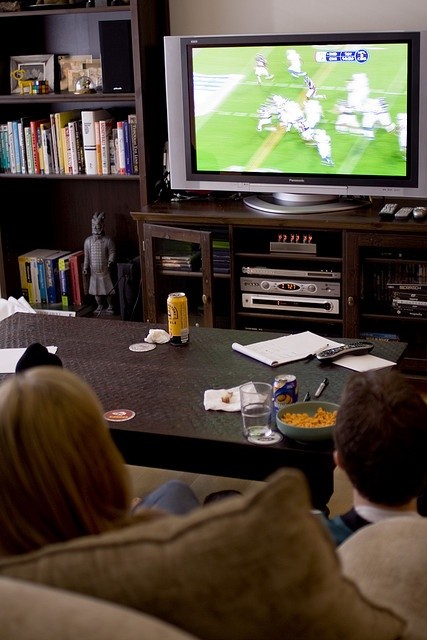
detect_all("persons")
[82,211,117,316]
[205,370,427,546]
[0,365,201,558]
[286,49,308,78]
[31,66,45,80]
[254,53,273,84]
[302,73,325,103]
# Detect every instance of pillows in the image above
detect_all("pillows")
[0,469,408,640]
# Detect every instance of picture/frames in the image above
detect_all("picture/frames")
[10,54,55,94]
[56,54,104,92]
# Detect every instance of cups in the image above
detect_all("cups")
[239,381,274,438]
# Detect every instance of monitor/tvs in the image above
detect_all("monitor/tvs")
[164,30,427,215]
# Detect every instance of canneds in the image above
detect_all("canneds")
[167,292,190,345]
[271,373,298,429]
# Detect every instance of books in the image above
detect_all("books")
[18,248,90,310]
[0,110,140,176]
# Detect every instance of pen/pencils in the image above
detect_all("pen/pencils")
[305,344,329,359]
[303,392,310,402]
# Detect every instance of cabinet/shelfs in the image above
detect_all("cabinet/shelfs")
[0,0,171,321]
[129,196,427,389]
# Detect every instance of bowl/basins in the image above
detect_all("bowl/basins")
[275,400,341,445]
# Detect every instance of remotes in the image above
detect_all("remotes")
[394,207,414,220]
[378,203,397,222]
[316,341,374,363]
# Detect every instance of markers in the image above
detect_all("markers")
[315,378,329,397]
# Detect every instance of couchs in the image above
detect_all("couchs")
[0,464,268,640]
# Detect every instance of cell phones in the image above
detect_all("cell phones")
[413,207,425,218]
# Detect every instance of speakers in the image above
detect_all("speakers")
[97,21,133,93]
